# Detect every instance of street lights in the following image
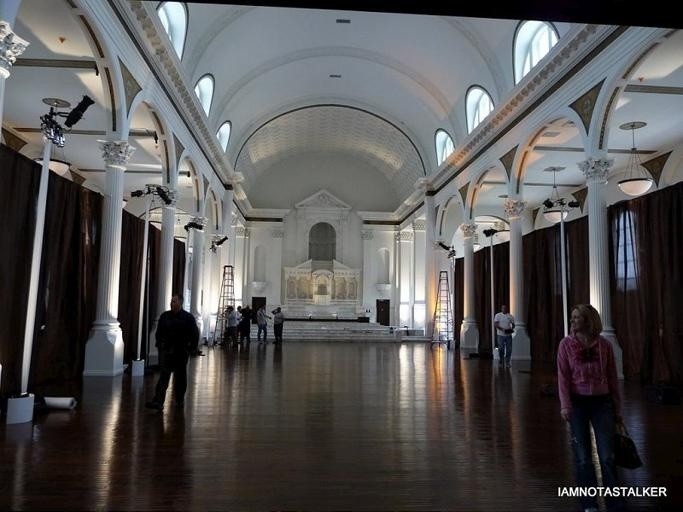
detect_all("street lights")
[441,244,459,346]
[180,214,207,359]
[206,232,228,351]
[481,225,497,360]
[539,195,581,340]
[6,92,96,425]
[128,182,173,377]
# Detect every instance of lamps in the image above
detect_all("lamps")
[33,98,71,177]
[149,194,162,230]
[543,167,568,223]
[174,218,187,242]
[616,121,654,196]
[122,199,127,209]
[496,195,510,241]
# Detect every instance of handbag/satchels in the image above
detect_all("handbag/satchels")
[608,423,644,470]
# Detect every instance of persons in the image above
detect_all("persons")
[219,302,284,346]
[557,304,623,512]
[145,293,199,412]
[494,304,515,370]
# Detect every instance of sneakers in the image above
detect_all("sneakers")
[498,364,511,369]
[146,401,164,410]
